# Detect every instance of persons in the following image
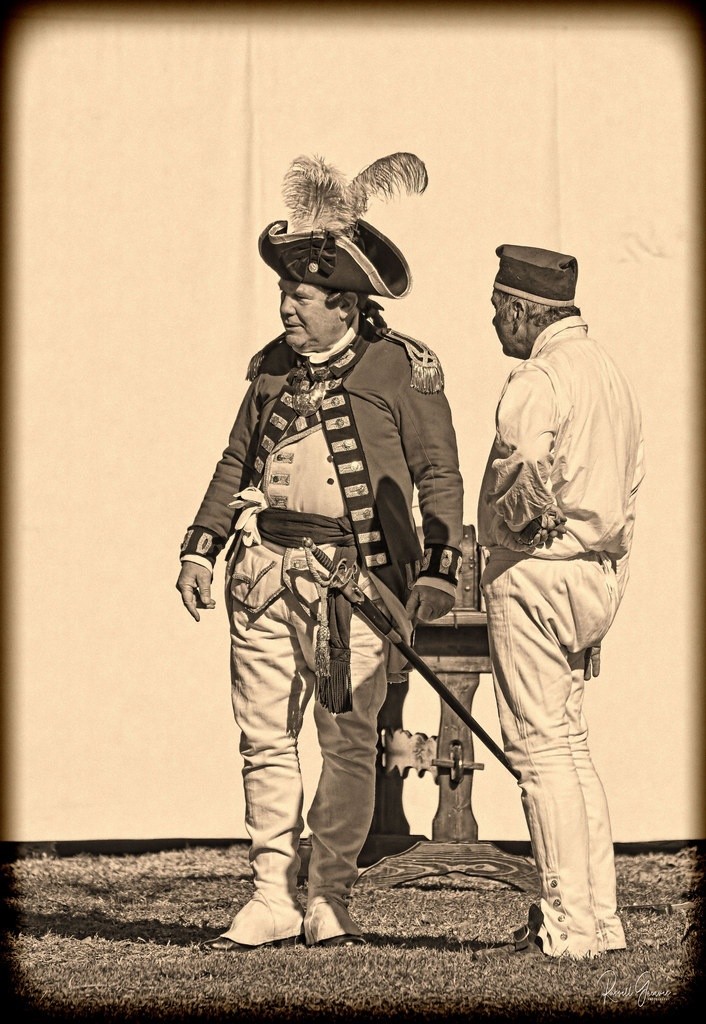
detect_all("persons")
[176,218,463,952]
[477,244,639,961]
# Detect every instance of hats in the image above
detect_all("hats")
[495,244,580,308]
[257,152,428,298]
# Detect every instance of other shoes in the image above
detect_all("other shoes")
[473,904,545,959]
[311,933,369,949]
[200,929,307,952]
